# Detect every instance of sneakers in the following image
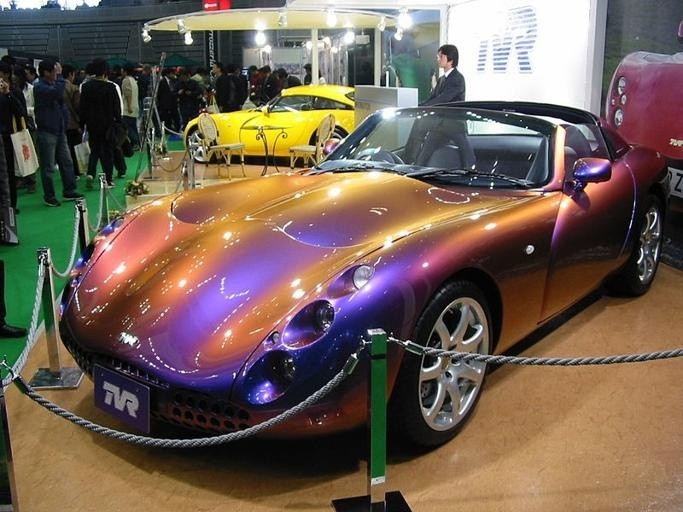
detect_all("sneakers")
[43,195,61,207]
[63,193,85,200]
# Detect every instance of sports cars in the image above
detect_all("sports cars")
[56,101,671,452]
[182,84,354,165]
[604,20,682,215]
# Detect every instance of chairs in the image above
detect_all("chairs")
[516,130,591,183]
[416,121,480,173]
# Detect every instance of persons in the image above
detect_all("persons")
[404,44,477,171]
[1,56,128,247]
[1,260,28,338]
[113,61,209,152]
[212,62,322,114]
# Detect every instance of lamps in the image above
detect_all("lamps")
[141,7,405,46]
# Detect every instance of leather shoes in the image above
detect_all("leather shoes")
[0,324,27,338]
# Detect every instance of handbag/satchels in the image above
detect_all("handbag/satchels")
[10,115,39,177]
[74,125,91,173]
